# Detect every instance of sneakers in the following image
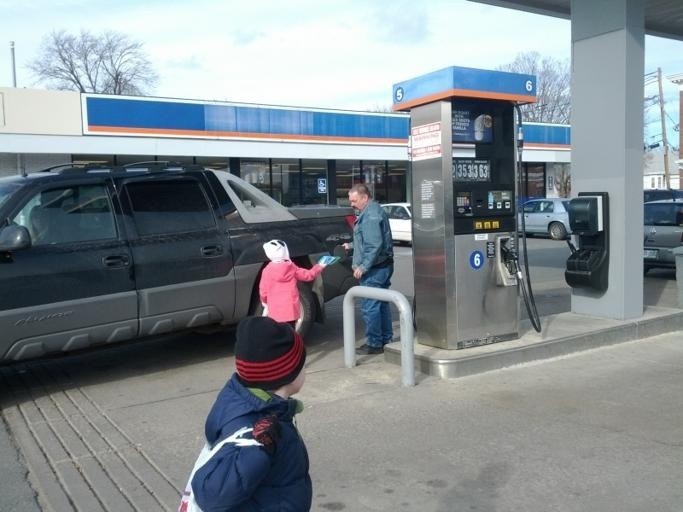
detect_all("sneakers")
[355,342,384,355]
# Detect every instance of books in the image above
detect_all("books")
[318,254,341,265]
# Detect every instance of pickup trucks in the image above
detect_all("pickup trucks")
[0,159,360,368]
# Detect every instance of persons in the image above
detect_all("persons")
[189,314,314,512]
[339,181,394,358]
[255,239,329,335]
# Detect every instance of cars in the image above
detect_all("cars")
[517,197,575,240]
[643,197,683,275]
[377,202,412,246]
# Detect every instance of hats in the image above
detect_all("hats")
[262,239,290,262]
[234,316,306,390]
